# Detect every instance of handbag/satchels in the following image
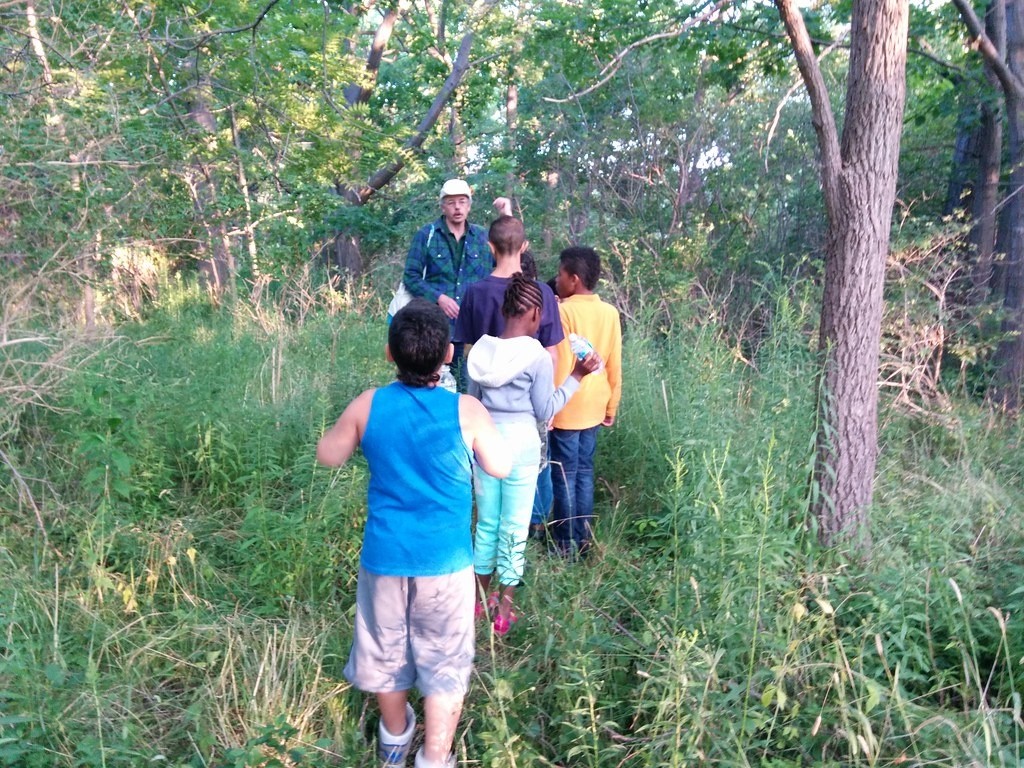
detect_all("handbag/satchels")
[385,223,435,323]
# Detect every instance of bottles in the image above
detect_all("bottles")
[437,365,456,394]
[569,333,605,376]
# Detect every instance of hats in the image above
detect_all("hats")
[440,179,471,202]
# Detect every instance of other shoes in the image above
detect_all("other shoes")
[474,590,501,619]
[494,610,523,635]
[531,523,545,540]
[378,700,415,768]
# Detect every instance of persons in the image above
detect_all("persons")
[316,299,513,768]
[554,246,623,563]
[452,215,565,539]
[467,271,603,638]
[402,179,513,366]
